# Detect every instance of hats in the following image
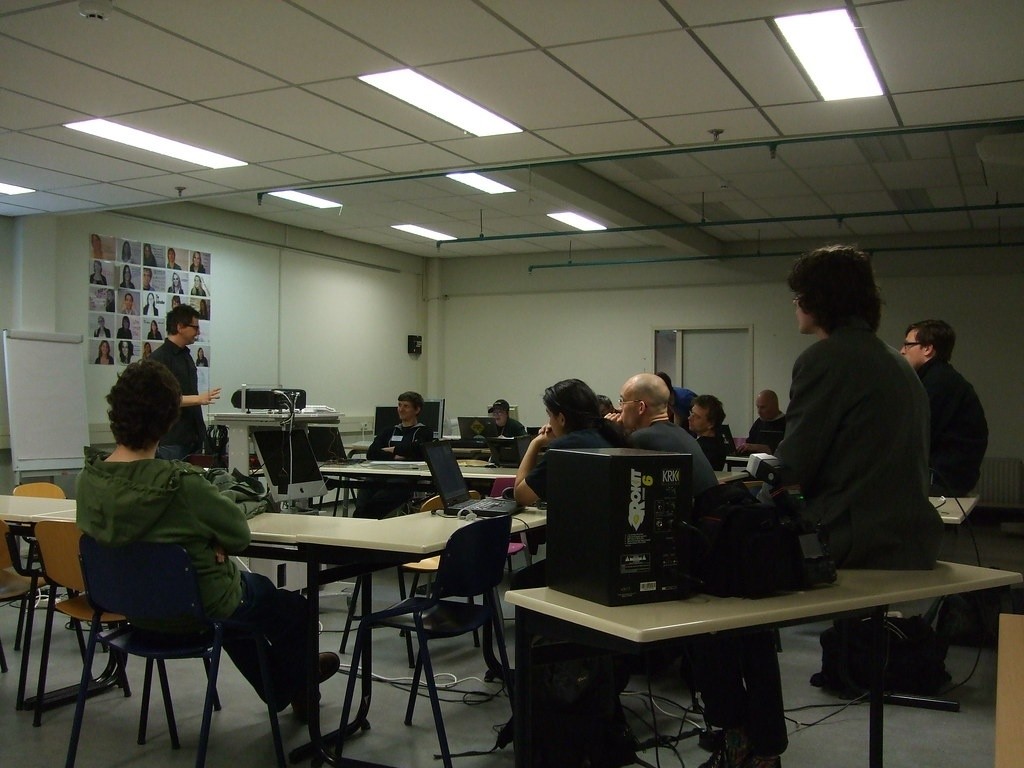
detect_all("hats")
[488,399,509,413]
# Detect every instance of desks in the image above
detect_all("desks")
[929,496,979,525]
[714,456,750,485]
[296,498,547,768]
[0,494,118,710]
[211,412,345,477]
[250,461,518,517]
[344,441,492,516]
[505,560,1022,768]
[30,509,379,764]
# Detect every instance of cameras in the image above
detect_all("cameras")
[746,453,838,590]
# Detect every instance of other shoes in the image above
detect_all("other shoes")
[292,692,307,723]
[318,652,339,683]
[810,669,822,688]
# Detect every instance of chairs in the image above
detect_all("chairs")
[0,483,287,768]
[334,490,513,768]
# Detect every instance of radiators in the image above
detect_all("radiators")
[977,458,1024,509]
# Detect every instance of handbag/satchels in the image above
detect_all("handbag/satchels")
[207,467,271,520]
[820,614,944,691]
[937,586,1024,645]
[694,486,838,595]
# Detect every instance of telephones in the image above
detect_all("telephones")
[408,335,422,354]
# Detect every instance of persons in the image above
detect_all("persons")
[511,378,633,586]
[897,319,988,499]
[653,372,700,439]
[74,357,340,712]
[132,304,221,461]
[351,390,435,519]
[89,233,209,367]
[481,400,526,438]
[604,372,719,487]
[687,393,735,472]
[774,244,945,571]
[736,390,788,468]
[595,391,620,418]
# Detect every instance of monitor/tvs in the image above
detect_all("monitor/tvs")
[373,406,401,437]
[251,427,328,511]
[417,398,445,439]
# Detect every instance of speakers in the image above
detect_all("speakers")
[546,448,696,607]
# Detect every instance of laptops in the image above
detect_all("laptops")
[421,440,525,517]
[458,416,498,443]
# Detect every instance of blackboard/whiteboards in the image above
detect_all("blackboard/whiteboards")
[3,328,92,471]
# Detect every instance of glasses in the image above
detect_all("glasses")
[618,398,648,408]
[690,411,701,421]
[187,324,200,331]
[905,341,932,349]
